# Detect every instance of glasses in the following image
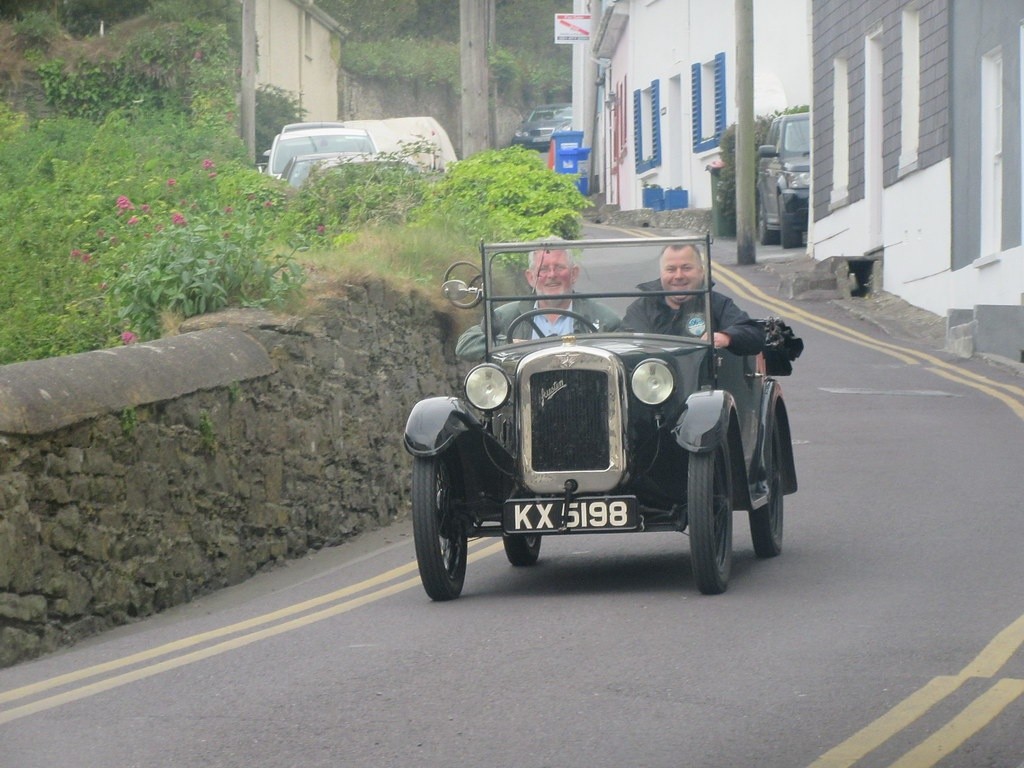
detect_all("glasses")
[534,264,571,273]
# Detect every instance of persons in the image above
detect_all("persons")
[454,235,622,362]
[608,241,769,357]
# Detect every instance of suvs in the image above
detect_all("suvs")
[756,112,810,249]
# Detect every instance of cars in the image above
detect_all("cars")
[403,232,799,600]
[513,103,573,150]
[262,122,420,192]
